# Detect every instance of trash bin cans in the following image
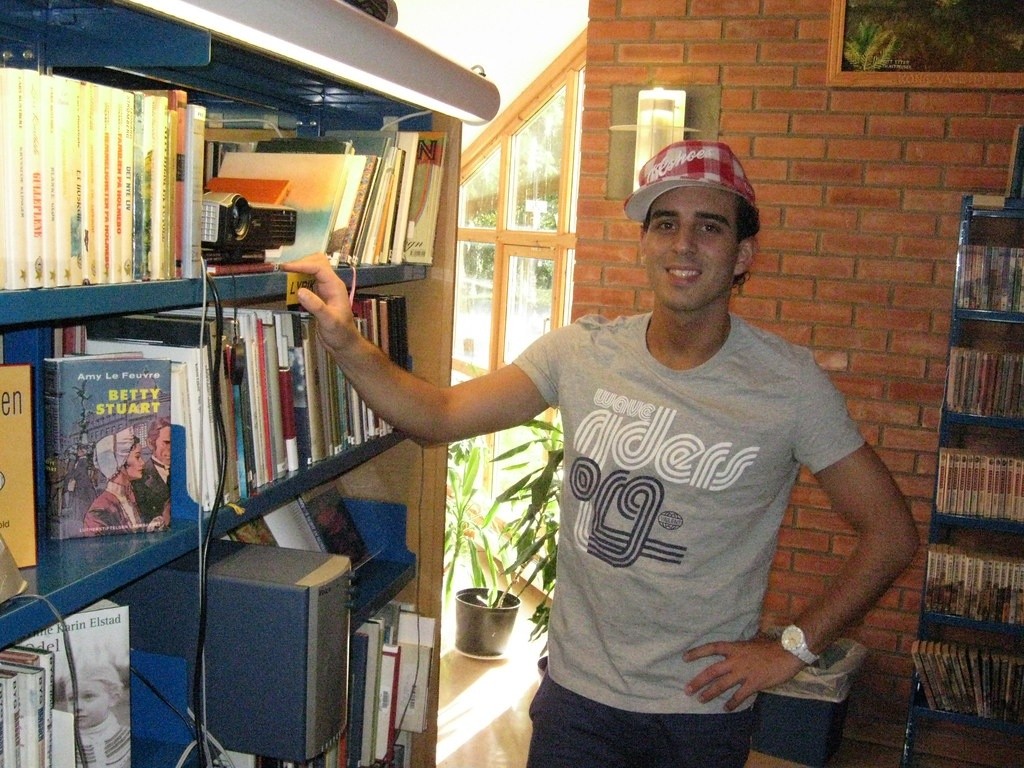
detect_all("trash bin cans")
[749,624,862,768]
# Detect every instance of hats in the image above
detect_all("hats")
[622,139,757,222]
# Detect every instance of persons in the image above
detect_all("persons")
[281,141,920,768]
[64,662,131,768]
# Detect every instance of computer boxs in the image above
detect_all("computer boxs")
[104,536,359,758]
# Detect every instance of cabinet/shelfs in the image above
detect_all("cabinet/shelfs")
[902,194,1024,768]
[0,2,497,767]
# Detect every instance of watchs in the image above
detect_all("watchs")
[781,623,819,665]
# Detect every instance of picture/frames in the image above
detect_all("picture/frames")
[825,0,1024,94]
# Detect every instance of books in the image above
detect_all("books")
[946,346,1024,417]
[203,111,449,269]
[957,243,1024,312]
[0,599,130,768]
[911,640,1024,724]
[0,295,409,603]
[225,481,435,768]
[936,447,1024,523]
[1,68,207,290]
[924,544,1024,624]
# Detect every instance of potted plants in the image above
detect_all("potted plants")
[454,406,566,660]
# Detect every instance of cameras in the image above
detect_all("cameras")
[198,189,297,263]
